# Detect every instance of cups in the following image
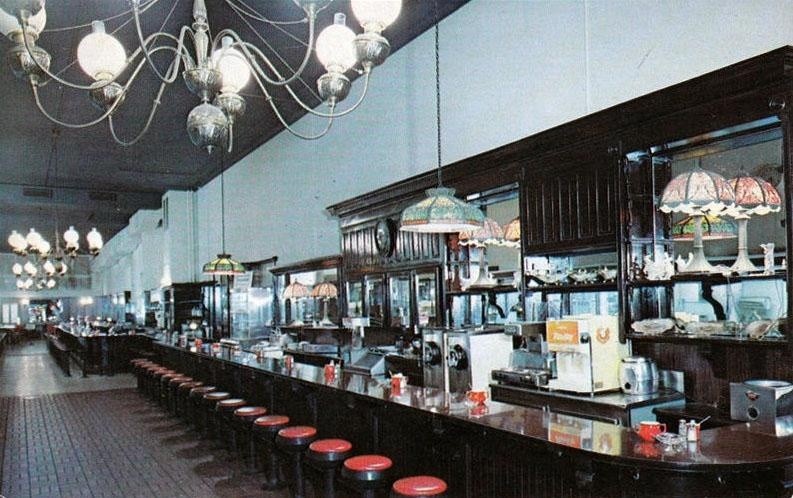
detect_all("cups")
[390,377,400,396]
[632,420,666,439]
[213,342,220,353]
[632,440,657,455]
[467,402,489,415]
[464,389,489,405]
[323,364,335,378]
[194,338,201,347]
[284,355,292,366]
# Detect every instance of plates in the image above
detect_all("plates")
[657,441,683,455]
[656,432,681,445]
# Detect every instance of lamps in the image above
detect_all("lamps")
[309,273,339,326]
[199,141,251,279]
[4,127,103,293]
[0,0,406,159]
[279,276,311,327]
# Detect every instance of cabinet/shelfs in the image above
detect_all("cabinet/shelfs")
[0,274,217,379]
[325,158,477,366]
[619,46,791,400]
[471,103,622,329]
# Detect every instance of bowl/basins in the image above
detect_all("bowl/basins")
[598,270,616,280]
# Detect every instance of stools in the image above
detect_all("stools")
[252,417,292,490]
[392,476,448,498]
[309,440,353,497]
[129,358,267,473]
[279,428,317,497]
[342,455,394,498]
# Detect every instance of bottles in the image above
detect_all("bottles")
[687,442,696,457]
[678,418,701,442]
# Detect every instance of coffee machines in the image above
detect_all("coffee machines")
[488,319,557,390]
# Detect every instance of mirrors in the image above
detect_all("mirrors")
[270,266,344,327]
